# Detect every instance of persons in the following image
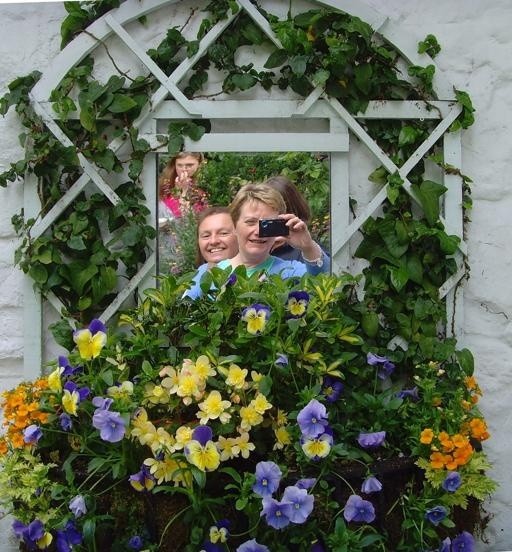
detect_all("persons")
[192,206,240,267]
[182,185,329,304]
[253,177,317,257]
[157,154,209,213]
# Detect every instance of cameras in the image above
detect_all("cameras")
[259,219,289,238]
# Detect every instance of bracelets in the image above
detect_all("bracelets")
[299,242,324,268]
